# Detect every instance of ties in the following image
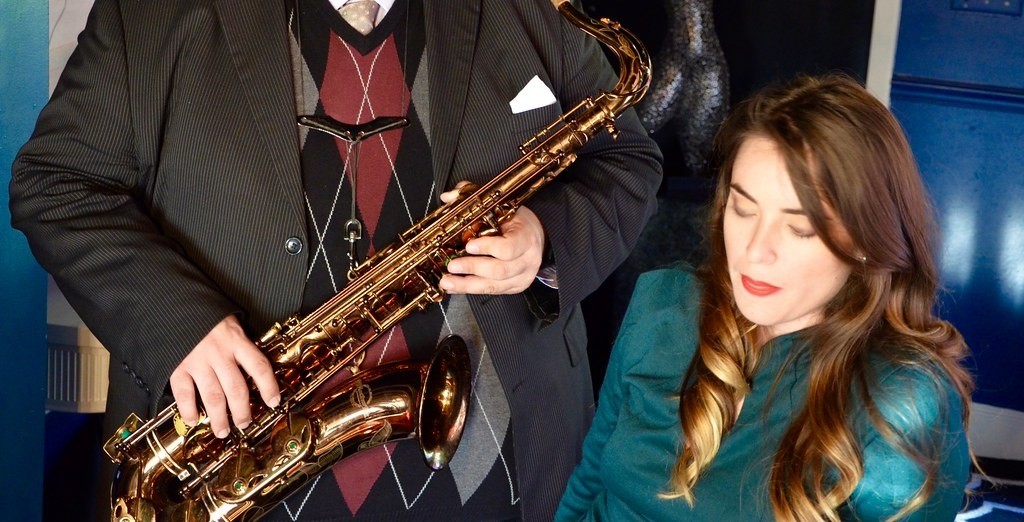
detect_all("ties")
[337,0,380,36]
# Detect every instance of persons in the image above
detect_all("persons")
[7,0,661,522]
[549,74,975,522]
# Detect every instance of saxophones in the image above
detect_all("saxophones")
[101,1,652,522]
